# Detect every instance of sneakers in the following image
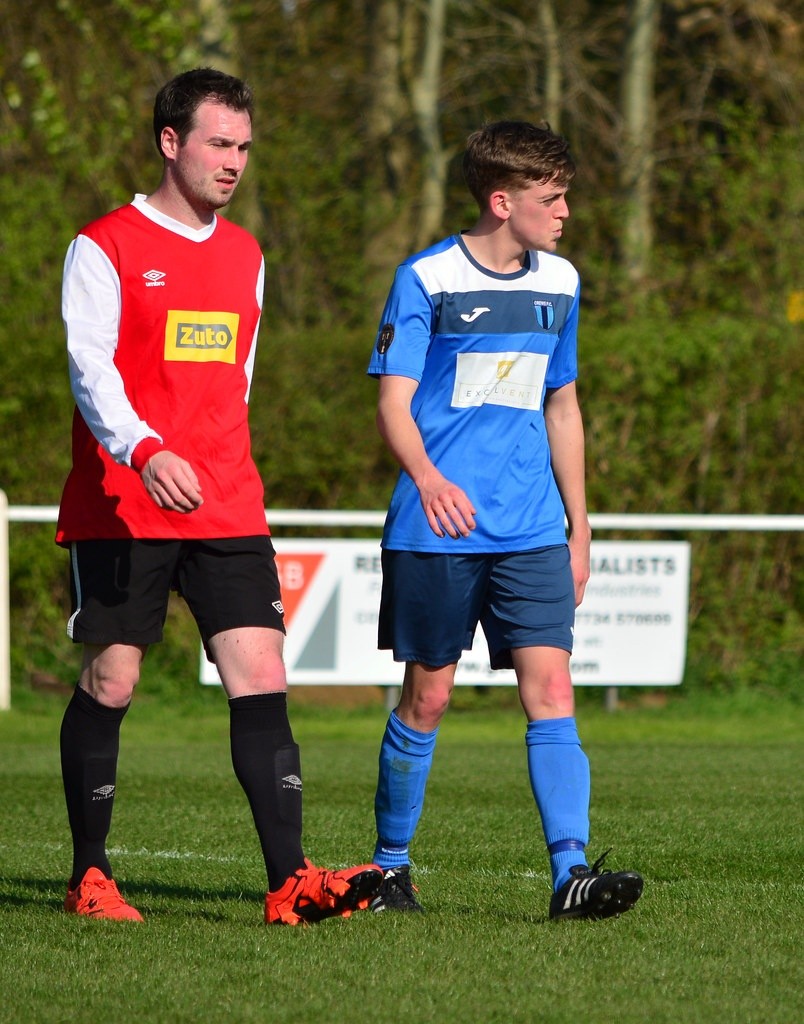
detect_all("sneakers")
[372,864,424,913]
[264,857,383,927]
[549,865,643,920]
[66,867,143,923]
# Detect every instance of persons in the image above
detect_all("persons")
[367,111,644,921]
[58,66,384,929]
